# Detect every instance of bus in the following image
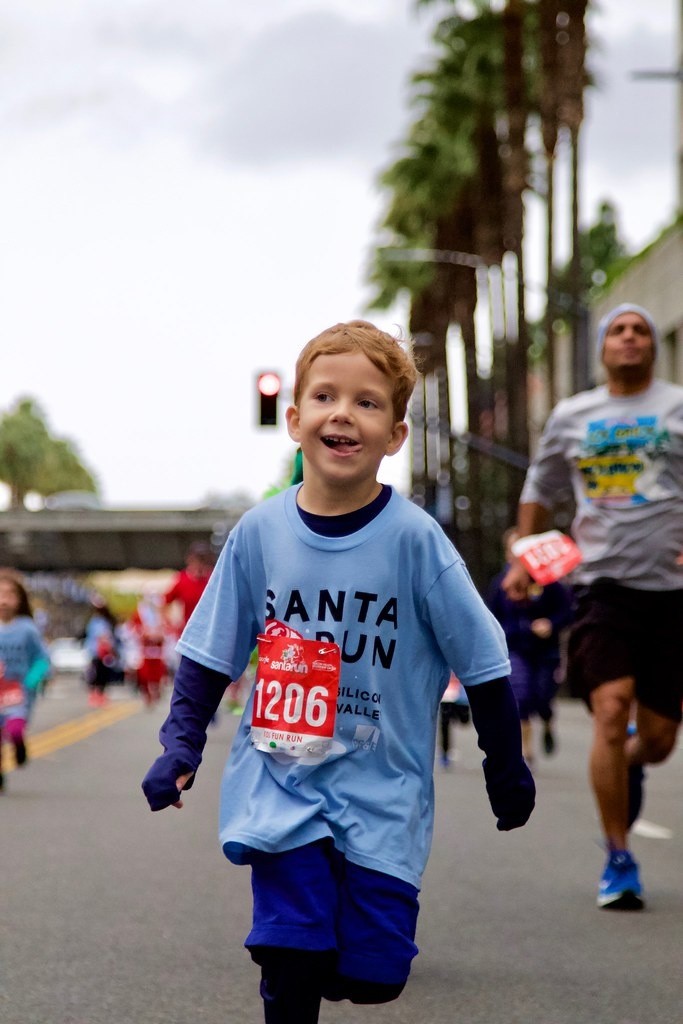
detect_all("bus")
[0,506,249,673]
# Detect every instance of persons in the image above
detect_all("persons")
[501,303,683,910]
[142,319,537,1024]
[439,526,554,766]
[0,553,244,795]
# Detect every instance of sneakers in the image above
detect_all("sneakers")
[625,763,644,829]
[597,849,644,910]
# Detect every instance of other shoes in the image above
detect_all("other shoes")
[543,731,553,752]
[441,755,449,769]
[15,742,26,764]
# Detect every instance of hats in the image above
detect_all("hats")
[596,302,658,365]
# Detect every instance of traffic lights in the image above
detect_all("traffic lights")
[256,371,281,425]
